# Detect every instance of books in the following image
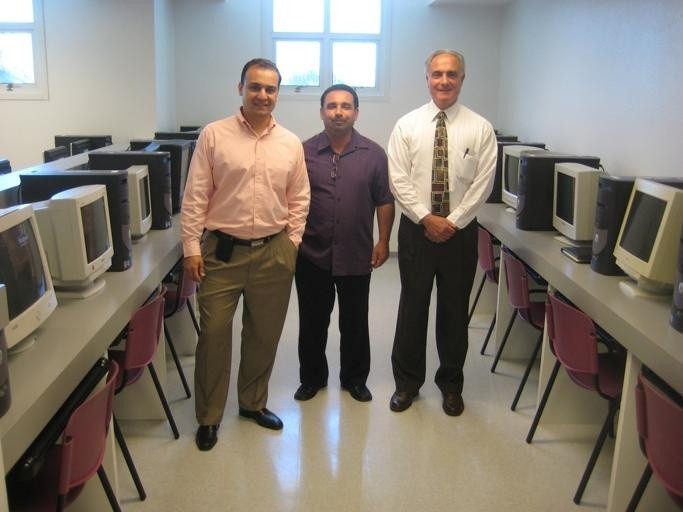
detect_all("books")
[559,242,597,269]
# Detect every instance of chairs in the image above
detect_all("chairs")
[25,357,129,512]
[466,227,500,356]
[489,244,548,410]
[524,290,621,507]
[160,257,202,399]
[624,364,682,509]
[107,284,182,502]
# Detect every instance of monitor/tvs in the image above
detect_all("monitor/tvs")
[0,159,12,176]
[501,145,545,213]
[70,139,91,156]
[553,163,610,248]
[0,204,58,356]
[26,185,114,298]
[44,146,68,163]
[613,177,683,300]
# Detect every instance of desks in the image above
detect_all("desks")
[0,212,198,512]
[475,202,677,507]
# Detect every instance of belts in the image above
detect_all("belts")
[212,230,276,248]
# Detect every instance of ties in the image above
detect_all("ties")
[431,112,448,218]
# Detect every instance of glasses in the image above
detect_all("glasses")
[330,152,339,177]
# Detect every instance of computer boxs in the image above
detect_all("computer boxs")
[591,175,683,275]
[485,141,545,203]
[496,135,518,142]
[20,169,132,272]
[0,327,11,418]
[88,126,204,230]
[669,221,683,334]
[55,136,111,150]
[515,150,600,230]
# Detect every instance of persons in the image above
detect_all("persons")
[292,82,394,403]
[386,49,498,418]
[178,57,311,452]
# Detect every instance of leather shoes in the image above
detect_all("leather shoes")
[195,424,219,451]
[294,381,327,400]
[239,408,282,430]
[442,392,463,416]
[390,389,418,411]
[340,383,371,402]
